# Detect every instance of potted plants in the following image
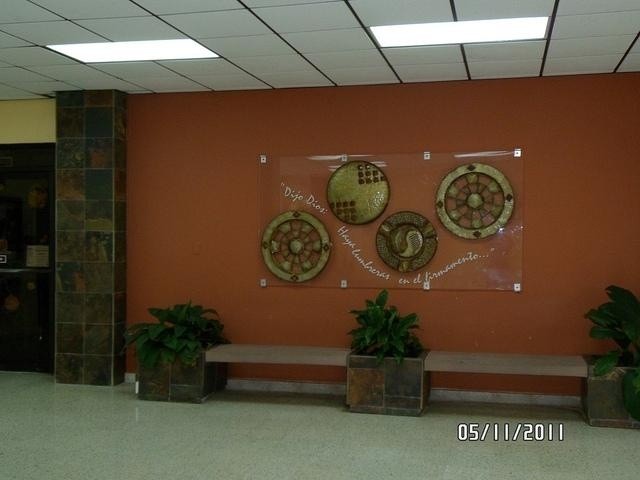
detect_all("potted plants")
[121,298,225,403]
[584,284,640,429]
[343,289,430,417]
